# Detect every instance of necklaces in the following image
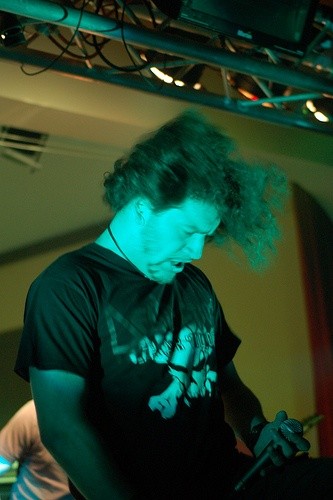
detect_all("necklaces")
[107,221,151,279]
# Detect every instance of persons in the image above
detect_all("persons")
[0,395,78,500]
[7,110,333,500]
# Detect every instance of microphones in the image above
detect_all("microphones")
[233,419,303,492]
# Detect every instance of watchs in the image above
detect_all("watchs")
[249,420,270,446]
